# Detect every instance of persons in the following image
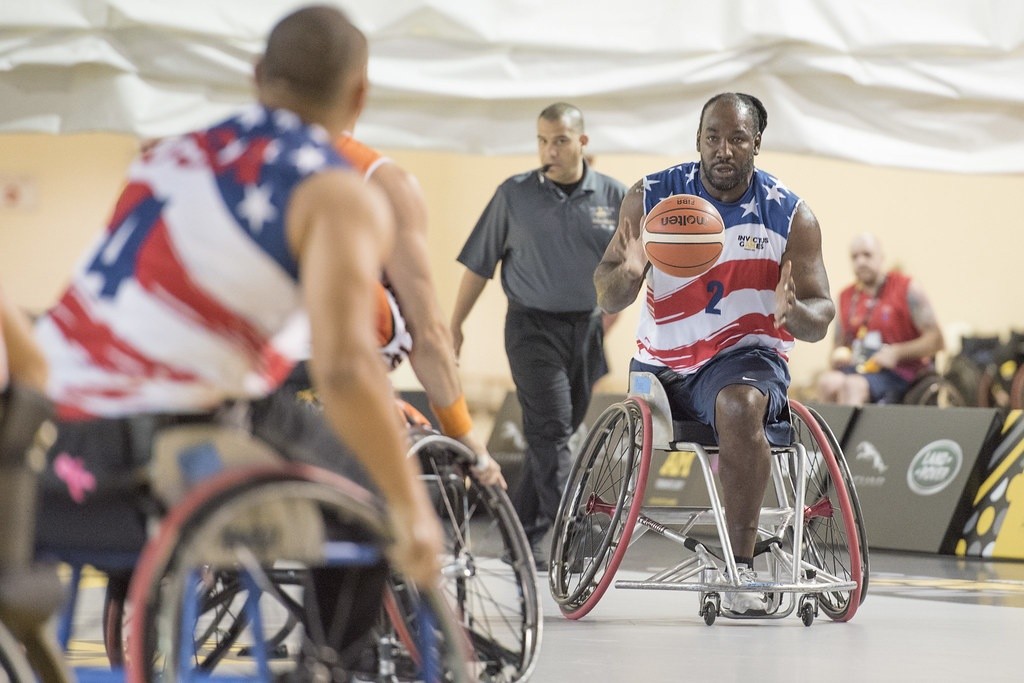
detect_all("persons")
[449,102,630,572]
[29,7,510,683]
[594,90,838,617]
[813,234,943,405]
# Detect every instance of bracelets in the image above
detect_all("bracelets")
[430,396,472,438]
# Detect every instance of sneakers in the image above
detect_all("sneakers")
[722,562,768,615]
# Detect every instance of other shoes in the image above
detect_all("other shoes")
[501,545,547,571]
[294,638,453,683]
[564,512,612,573]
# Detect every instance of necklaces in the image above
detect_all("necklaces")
[847,270,890,337]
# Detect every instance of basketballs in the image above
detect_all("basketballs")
[641,191,727,280]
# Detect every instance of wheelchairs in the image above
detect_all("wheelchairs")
[551,396,870,624]
[0,361,543,683]
